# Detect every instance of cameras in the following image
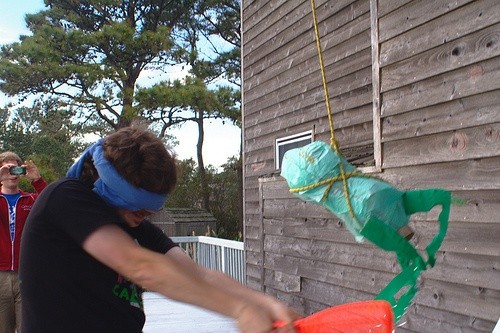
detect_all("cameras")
[9,166,27,175]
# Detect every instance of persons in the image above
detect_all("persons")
[0,151,47,333]
[19,125,304,333]
